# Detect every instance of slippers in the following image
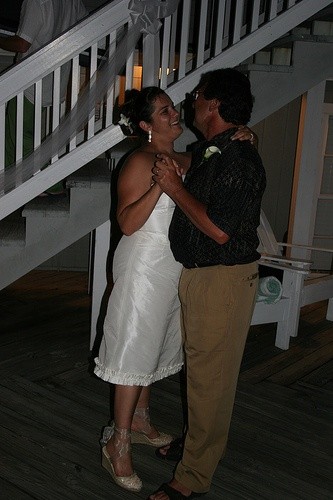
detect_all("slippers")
[35,191,67,201]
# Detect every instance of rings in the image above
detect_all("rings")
[154,170,159,174]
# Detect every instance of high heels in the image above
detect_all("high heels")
[100,425,143,492]
[102,407,174,447]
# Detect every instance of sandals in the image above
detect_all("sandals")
[155,436,184,460]
[148,477,208,500]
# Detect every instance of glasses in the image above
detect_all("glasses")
[192,89,219,101]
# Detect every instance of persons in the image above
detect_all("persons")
[0,0,91,198]
[147,68,266,500]
[93,85,260,491]
